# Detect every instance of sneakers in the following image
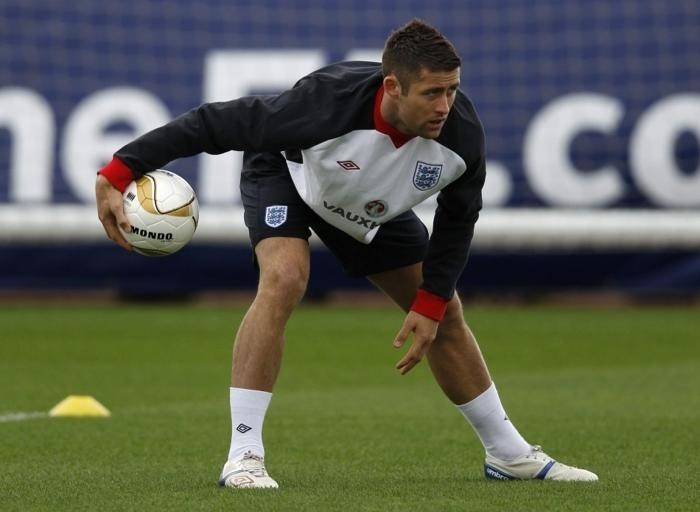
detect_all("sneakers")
[217,450,279,491]
[484,445,600,483]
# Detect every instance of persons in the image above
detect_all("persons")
[93,17,600,489]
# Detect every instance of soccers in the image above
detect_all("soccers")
[117,169,199,257]
